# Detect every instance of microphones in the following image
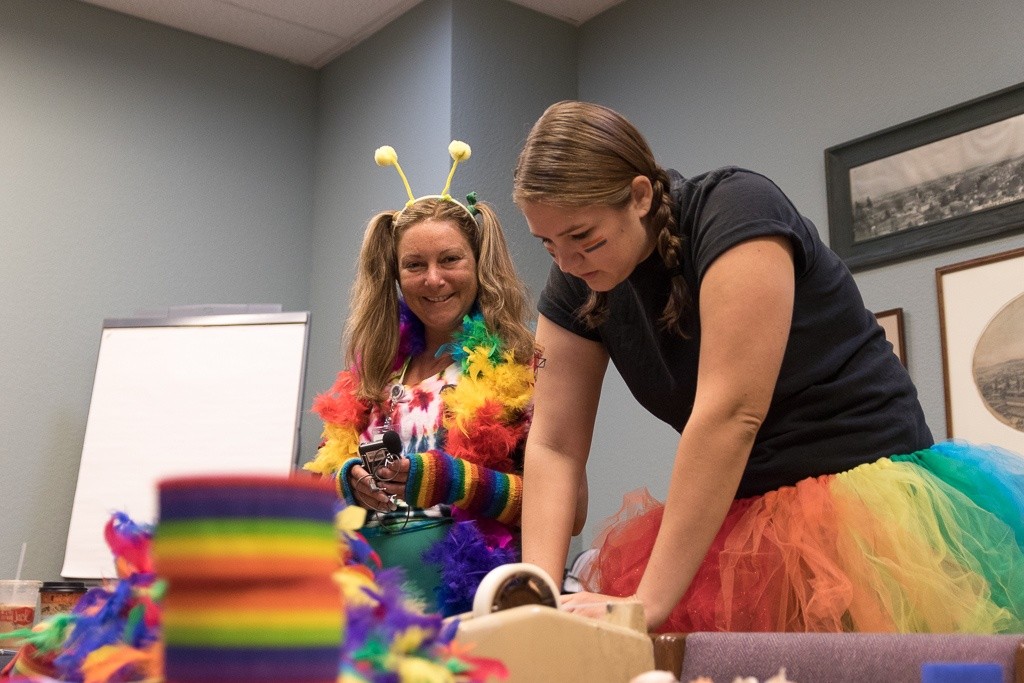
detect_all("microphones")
[382,432,403,460]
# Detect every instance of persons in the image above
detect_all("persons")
[511,96,1024,635]
[301,138,590,613]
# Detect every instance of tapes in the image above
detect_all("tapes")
[472,562,563,619]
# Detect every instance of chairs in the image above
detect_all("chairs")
[654,632,1024,683]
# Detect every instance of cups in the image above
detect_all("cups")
[39,580,88,622]
[0,579,42,649]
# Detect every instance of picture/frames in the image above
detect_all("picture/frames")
[824,81,1024,270]
[873,307,906,371]
[935,247,1024,455]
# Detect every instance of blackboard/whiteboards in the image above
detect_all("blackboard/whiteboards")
[60,308,313,586]
